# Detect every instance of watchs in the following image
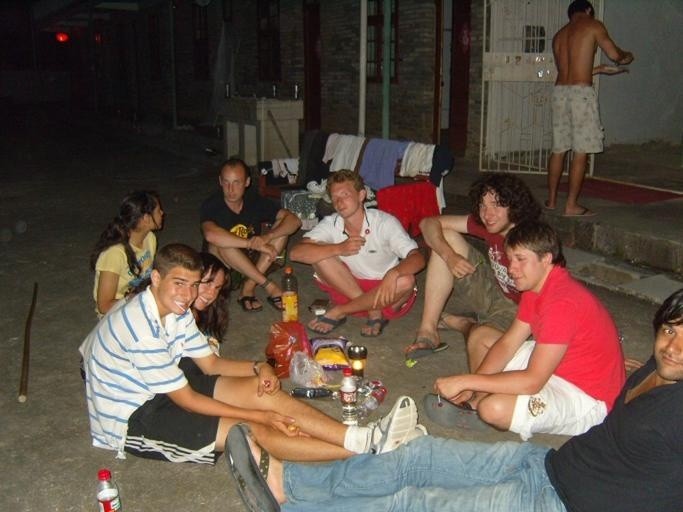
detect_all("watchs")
[252,360,261,376]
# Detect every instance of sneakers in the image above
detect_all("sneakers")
[363,395,428,455]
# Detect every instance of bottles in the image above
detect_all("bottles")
[225,83,231,98]
[293,82,299,100]
[347,345,367,392]
[271,83,278,98]
[356,387,387,422]
[281,266,299,324]
[339,368,360,427]
[290,386,337,400]
[95,469,123,512]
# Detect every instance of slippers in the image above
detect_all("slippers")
[236,295,477,358]
[544,199,557,210]
[563,208,598,218]
[224,423,284,512]
[423,393,490,430]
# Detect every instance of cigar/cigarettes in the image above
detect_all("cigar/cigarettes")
[275,256,284,260]
[474,261,481,270]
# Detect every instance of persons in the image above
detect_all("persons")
[86,244,434,463]
[204,160,302,314]
[291,174,425,338]
[545,2,632,215]
[79,254,232,383]
[407,174,567,356]
[92,194,167,324]
[423,221,629,440]
[224,284,682,512]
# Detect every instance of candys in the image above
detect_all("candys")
[474,260,484,267]
[288,424,296,433]
[363,228,370,239]
[437,395,443,407]
[265,382,270,387]
